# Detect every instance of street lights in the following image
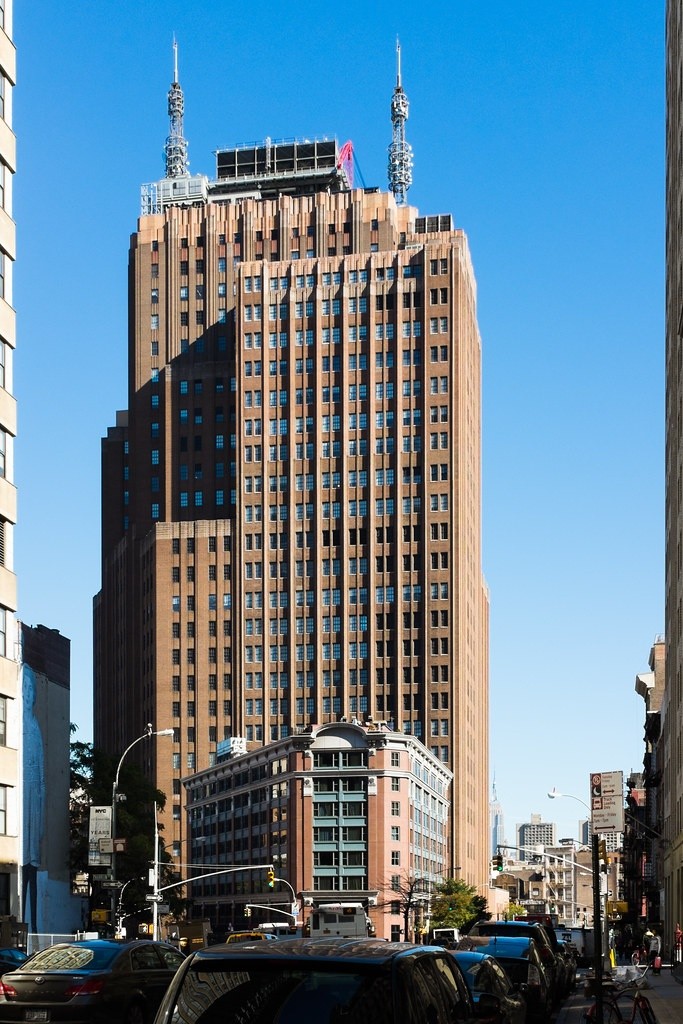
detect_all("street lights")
[118,876,146,938]
[273,878,297,927]
[558,839,611,972]
[108,729,174,926]
[403,866,461,942]
[547,791,602,994]
[148,836,207,941]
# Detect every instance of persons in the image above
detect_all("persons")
[615,927,662,975]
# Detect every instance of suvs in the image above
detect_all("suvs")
[152,938,478,1024]
[225,929,279,943]
[468,918,568,1012]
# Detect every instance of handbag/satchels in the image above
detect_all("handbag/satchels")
[655,956,662,968]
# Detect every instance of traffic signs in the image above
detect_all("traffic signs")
[591,796,624,835]
[100,881,125,889]
[590,771,623,796]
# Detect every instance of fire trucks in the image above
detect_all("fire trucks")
[514,911,558,930]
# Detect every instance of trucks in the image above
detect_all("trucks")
[553,926,605,968]
[253,923,302,941]
[309,902,375,939]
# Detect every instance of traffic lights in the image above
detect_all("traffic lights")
[266,872,274,888]
[597,839,606,860]
[550,907,555,913]
[448,901,454,911]
[496,855,503,871]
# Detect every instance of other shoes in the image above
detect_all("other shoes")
[653,972,660,975]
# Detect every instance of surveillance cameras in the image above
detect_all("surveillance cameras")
[120,796,127,803]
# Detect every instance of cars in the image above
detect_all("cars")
[0,945,28,976]
[0,938,188,1024]
[455,935,555,1020]
[553,942,571,1000]
[447,947,529,1024]
[560,943,577,990]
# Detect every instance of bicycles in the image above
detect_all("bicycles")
[581,960,658,1024]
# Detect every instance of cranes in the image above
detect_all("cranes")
[338,138,353,189]
